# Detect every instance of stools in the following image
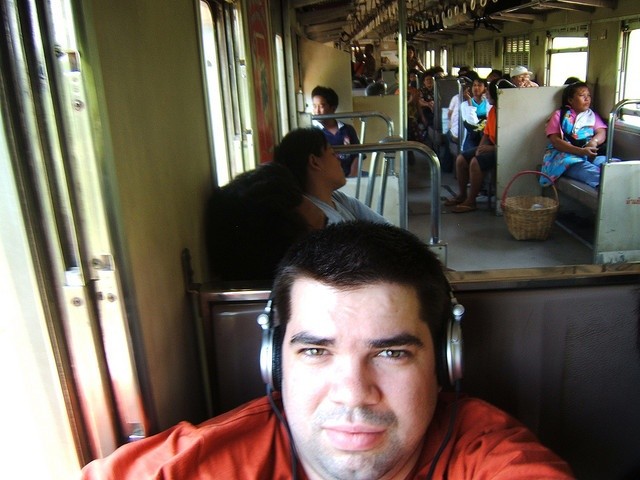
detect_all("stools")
[477,160,497,211]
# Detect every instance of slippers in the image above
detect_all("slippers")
[446,199,463,207]
[451,204,478,212]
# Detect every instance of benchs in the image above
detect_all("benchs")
[537,154,601,253]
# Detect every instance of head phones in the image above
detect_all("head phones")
[257,291,466,392]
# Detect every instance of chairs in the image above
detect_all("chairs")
[416,75,423,91]
[433,75,459,157]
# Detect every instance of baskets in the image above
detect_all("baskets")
[501,170,560,241]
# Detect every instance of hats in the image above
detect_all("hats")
[510,66,534,79]
[458,67,468,73]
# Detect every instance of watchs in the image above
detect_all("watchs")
[592,137,600,145]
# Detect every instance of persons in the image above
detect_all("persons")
[545,82,621,188]
[418,74,435,128]
[390,70,417,128]
[274,128,395,228]
[460,78,488,189]
[77,220,574,480]
[201,158,331,288]
[352,43,398,95]
[408,70,420,87]
[307,86,367,176]
[426,66,444,77]
[486,69,502,81]
[443,78,512,214]
[510,66,540,87]
[447,77,473,144]
[407,45,425,72]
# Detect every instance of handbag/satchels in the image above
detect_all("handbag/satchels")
[470,118,487,142]
[574,136,607,156]
